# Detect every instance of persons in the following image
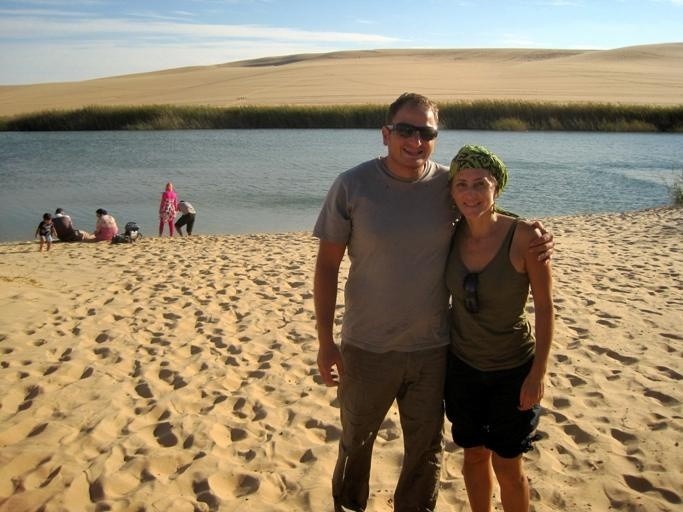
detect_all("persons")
[436,143,554,509]
[155,180,176,237]
[174,197,197,237]
[32,212,57,252]
[92,208,119,241]
[306,90,556,509]
[49,206,98,243]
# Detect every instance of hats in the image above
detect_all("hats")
[448,144,507,199]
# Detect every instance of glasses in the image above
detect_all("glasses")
[464,273,479,313]
[386,122,437,141]
